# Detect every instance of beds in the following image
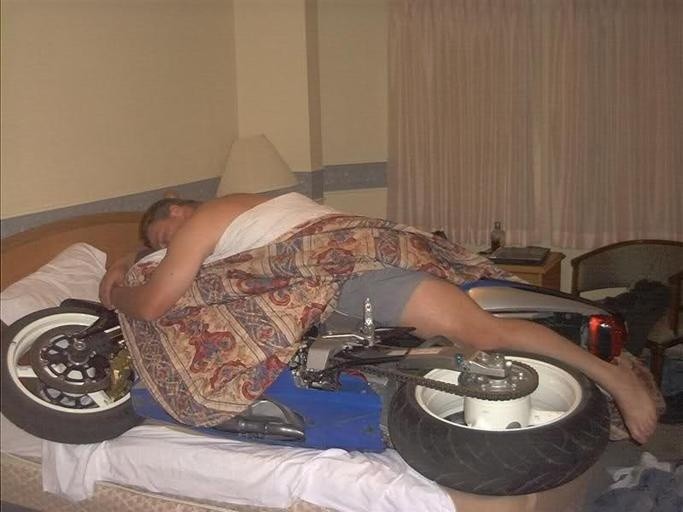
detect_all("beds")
[0,191,681,510]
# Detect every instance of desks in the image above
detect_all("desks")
[474,246,566,292]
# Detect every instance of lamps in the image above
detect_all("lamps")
[215,134,298,200]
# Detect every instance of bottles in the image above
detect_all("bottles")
[490,222,506,250]
[360,297,376,347]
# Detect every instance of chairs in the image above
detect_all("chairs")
[570,238,682,423]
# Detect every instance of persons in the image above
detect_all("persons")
[98,193,659,446]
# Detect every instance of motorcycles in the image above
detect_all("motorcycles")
[2,278,681,496]
